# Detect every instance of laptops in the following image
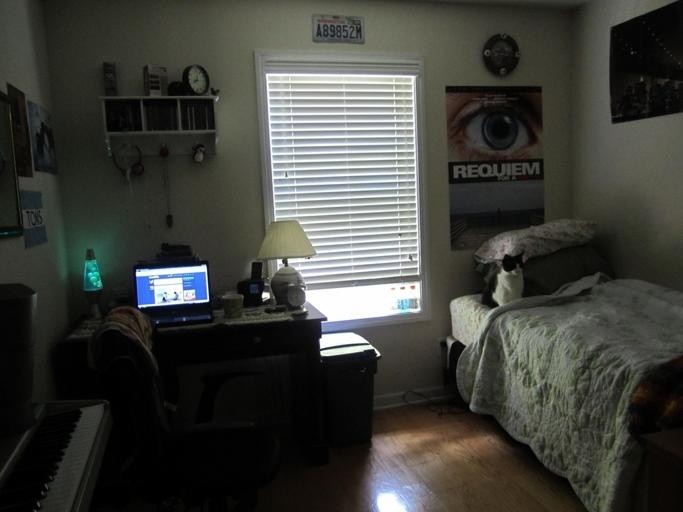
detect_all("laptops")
[132,260,213,328]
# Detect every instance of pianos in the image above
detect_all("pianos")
[0,399,113,512]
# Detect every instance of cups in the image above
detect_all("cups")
[221,293,244,319]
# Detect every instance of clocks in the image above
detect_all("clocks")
[182,64,209,95]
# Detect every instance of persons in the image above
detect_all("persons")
[445,91,544,165]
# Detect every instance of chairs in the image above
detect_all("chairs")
[89,305,274,512]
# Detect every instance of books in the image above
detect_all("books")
[103,97,214,134]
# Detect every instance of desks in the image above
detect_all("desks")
[55,298,327,471]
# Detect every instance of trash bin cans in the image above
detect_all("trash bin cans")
[319,332,383,443]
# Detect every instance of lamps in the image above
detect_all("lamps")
[256,221,316,315]
[82,248,104,322]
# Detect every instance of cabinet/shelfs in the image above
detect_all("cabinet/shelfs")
[95,94,218,161]
[639,425,681,511]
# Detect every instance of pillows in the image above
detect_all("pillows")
[473,215,599,269]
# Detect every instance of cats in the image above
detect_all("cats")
[482,251,524,309]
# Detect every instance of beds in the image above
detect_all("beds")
[444,277,682,511]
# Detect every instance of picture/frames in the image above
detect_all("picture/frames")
[0,82,34,239]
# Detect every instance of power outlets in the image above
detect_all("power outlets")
[437,336,447,350]
